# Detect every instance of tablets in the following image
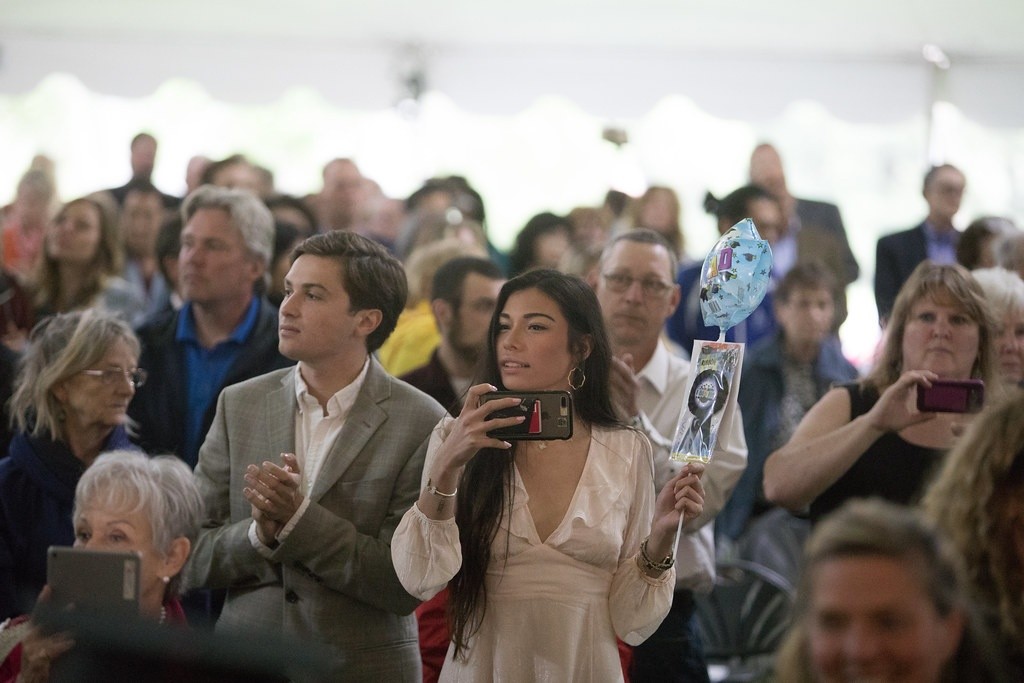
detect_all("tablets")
[47,547,142,621]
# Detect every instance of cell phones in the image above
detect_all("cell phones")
[480,389,573,441]
[917,380,987,414]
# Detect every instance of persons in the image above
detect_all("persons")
[0,132,1024,683]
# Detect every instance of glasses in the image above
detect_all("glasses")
[78,368,150,388]
[602,273,676,299]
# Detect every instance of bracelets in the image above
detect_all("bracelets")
[427,477,458,501]
[640,535,675,572]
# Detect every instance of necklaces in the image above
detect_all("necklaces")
[536,423,591,449]
